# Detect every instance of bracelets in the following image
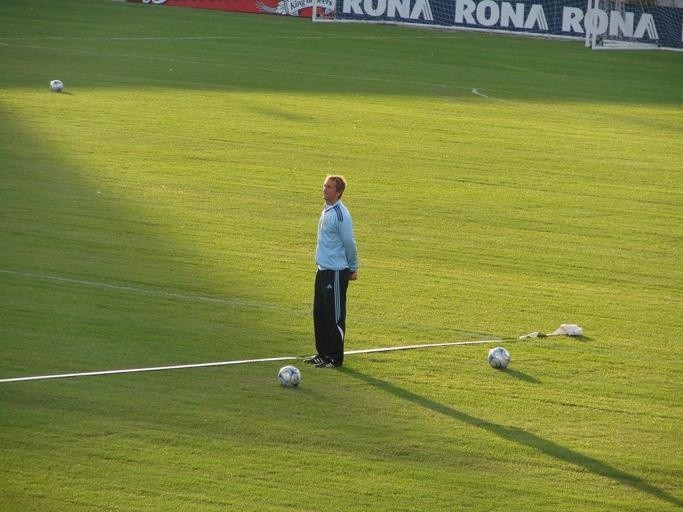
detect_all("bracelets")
[348,272,355,274]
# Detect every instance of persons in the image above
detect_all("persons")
[303,174,358,368]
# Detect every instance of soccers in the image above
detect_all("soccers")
[488,348,510,368]
[277,366,300,387]
[50,80,64,93]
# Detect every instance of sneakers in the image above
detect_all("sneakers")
[313,360,342,369]
[303,355,330,363]
